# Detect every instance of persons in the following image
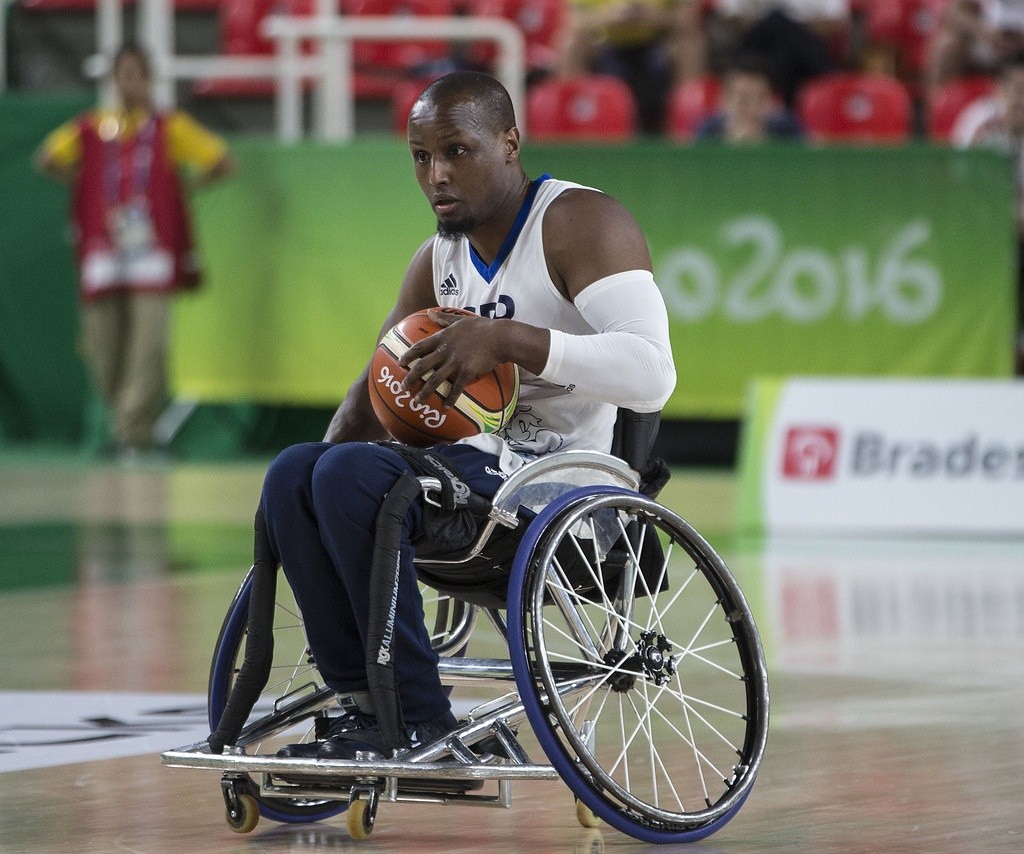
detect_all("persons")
[198,0,1024,158]
[258,70,676,771]
[32,42,229,454]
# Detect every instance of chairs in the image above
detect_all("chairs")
[216,2,1023,148]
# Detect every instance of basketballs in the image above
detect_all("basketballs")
[367,307,521,447]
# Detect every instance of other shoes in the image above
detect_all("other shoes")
[275,710,482,792]
[311,712,356,744]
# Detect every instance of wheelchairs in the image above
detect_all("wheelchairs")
[156,389,771,844]
